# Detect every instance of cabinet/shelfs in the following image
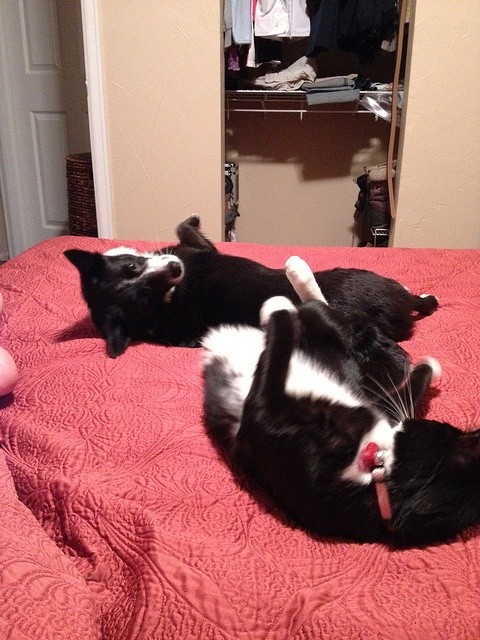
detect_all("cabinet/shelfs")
[97,0,479,248]
[224,87,400,123]
[370,226,390,247]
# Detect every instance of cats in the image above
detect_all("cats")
[197,254,479,549]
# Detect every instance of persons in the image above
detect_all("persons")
[0,291,19,397]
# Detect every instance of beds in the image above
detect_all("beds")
[0,236,479,640]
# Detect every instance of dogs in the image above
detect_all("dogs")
[61,213,441,358]
